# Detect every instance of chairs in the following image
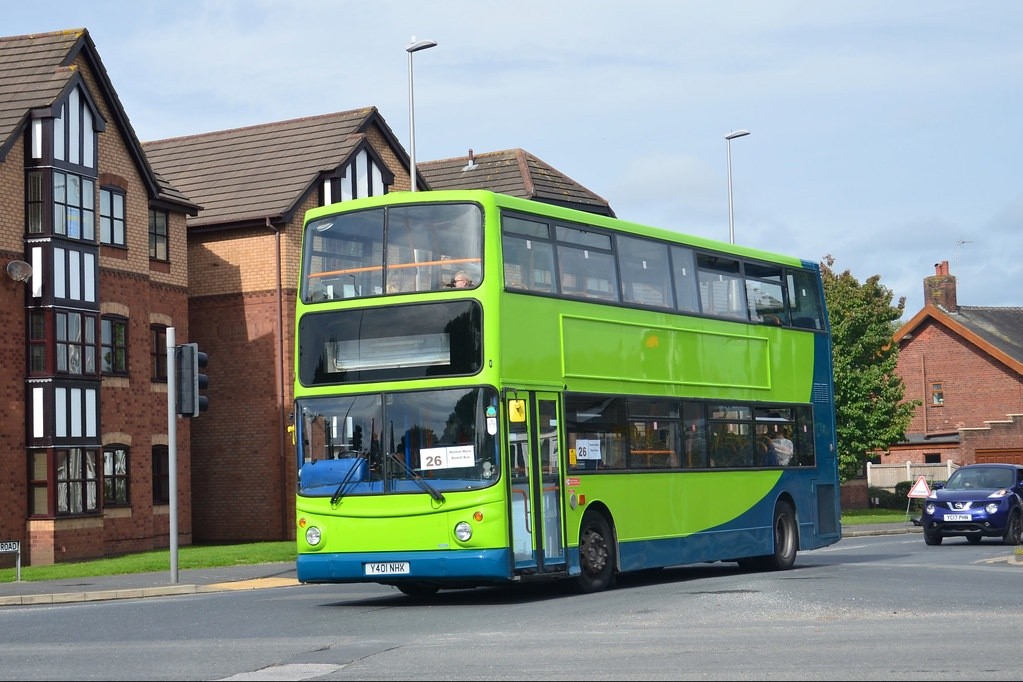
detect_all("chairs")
[511,282,781,324]
[711,434,778,466]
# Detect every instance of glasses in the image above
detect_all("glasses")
[454,279,463,283]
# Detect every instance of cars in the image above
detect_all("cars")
[920,464,1023,547]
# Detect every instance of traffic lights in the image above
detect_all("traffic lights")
[639,329,666,382]
[178,343,210,418]
[347,431,362,452]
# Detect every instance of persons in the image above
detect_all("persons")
[361,408,405,476]
[757,430,793,465]
[454,270,475,288]
[386,269,404,293]
[308,272,328,301]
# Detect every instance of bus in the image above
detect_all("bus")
[284,188,844,598]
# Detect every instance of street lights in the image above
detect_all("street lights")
[408,39,439,189]
[724,129,748,244]
